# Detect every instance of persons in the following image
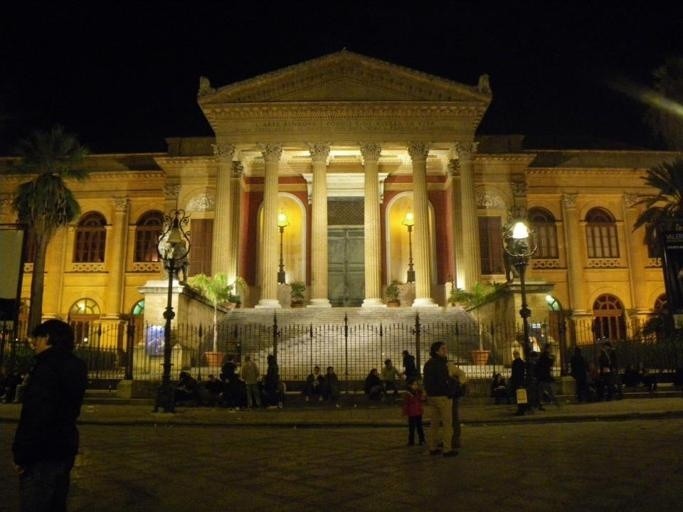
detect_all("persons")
[151,354,280,413]
[490,342,558,416]
[362,341,466,456]
[304,367,328,402]
[570,341,616,402]
[324,367,341,408]
[8,319,88,512]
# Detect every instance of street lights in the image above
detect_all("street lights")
[277,213,289,284]
[402,212,416,284]
[151,208,191,414]
[497,203,539,408]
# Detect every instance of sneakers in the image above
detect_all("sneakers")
[432,447,444,456]
[443,450,460,458]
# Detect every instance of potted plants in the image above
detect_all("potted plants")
[189,271,252,368]
[288,279,305,308]
[383,280,402,307]
[444,279,499,365]
